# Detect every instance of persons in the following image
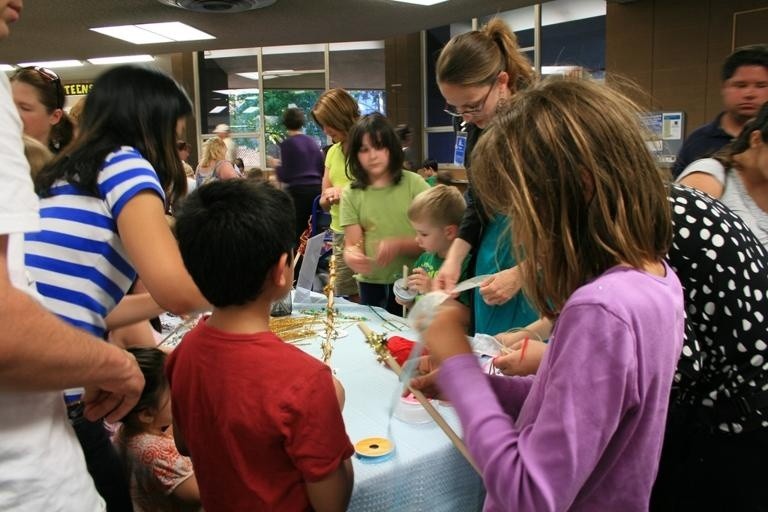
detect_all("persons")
[339,113,432,317]
[670,44,768,179]
[432,17,559,344]
[8,68,75,156]
[212,124,236,164]
[20,131,53,180]
[249,169,262,178]
[505,178,768,512]
[423,159,438,188]
[195,139,239,187]
[675,102,768,252]
[268,108,325,230]
[28,61,215,511]
[401,78,685,512]
[167,178,353,512]
[176,141,194,177]
[118,345,202,511]
[397,184,475,339]
[0,0,148,512]
[311,88,362,304]
[393,123,415,173]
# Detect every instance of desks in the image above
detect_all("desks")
[154,284,501,512]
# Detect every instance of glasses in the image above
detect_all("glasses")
[442,66,503,117]
[18,64,62,82]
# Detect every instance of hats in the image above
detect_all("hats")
[213,123,230,135]
